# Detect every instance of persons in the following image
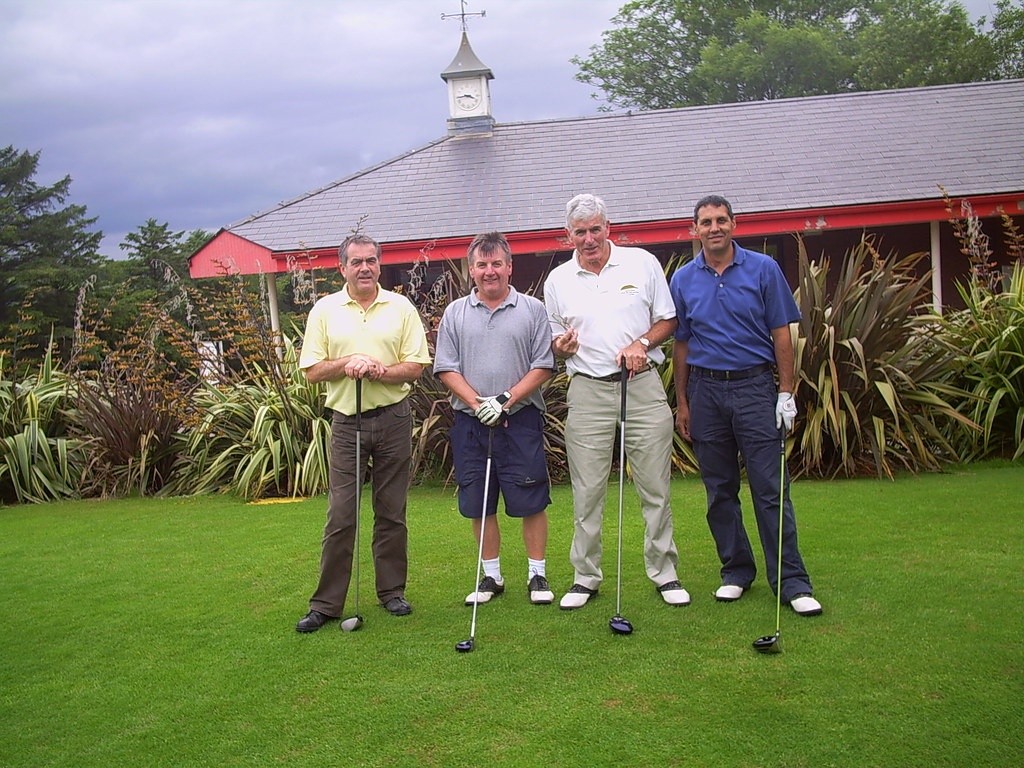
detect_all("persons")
[433,232,558,605]
[296,235,431,632]
[670,195,822,617]
[543,195,691,609]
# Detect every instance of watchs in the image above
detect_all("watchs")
[638,336,651,347]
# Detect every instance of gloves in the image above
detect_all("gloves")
[474,391,511,428]
[775,392,798,431]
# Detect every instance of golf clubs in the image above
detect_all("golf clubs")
[609,355,633,635]
[454,423,496,652]
[751,418,787,649]
[338,377,365,634]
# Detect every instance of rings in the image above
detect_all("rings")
[373,364,376,368]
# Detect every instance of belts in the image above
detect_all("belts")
[575,361,656,382]
[349,407,385,418]
[691,362,771,381]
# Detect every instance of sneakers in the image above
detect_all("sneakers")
[384,597,412,616]
[560,584,598,609]
[465,575,505,606]
[715,584,744,603]
[528,567,554,604]
[296,610,338,633]
[790,593,822,617]
[656,581,690,605]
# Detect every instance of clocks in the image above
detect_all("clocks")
[455,84,481,110]
